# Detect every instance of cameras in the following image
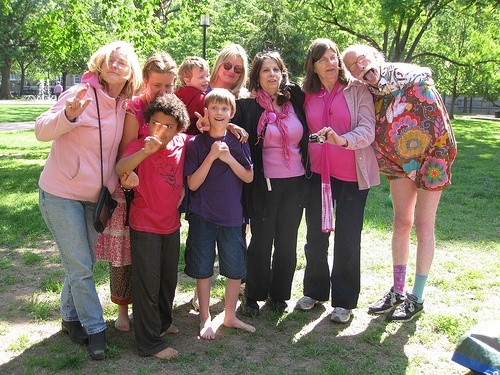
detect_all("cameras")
[309,134,326,145]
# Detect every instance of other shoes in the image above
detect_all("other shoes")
[240,296,259,316]
[268,296,289,314]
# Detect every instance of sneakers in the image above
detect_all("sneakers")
[88,328,106,360]
[330,306,352,324]
[368,286,406,314]
[295,295,319,311]
[385,291,424,323]
[62,319,88,345]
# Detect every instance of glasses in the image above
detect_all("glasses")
[317,56,340,64]
[223,62,242,74]
[350,52,368,72]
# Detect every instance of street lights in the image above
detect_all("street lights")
[200,11,211,60]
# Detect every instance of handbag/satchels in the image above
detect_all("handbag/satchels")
[93,185,118,233]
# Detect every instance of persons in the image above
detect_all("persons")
[183,88,257,339]
[342,44,458,321]
[191,43,248,309]
[54,81,63,101]
[176,56,211,135]
[195,51,309,316]
[115,94,249,360]
[296,38,381,323]
[36,42,143,359]
[95,52,178,331]
